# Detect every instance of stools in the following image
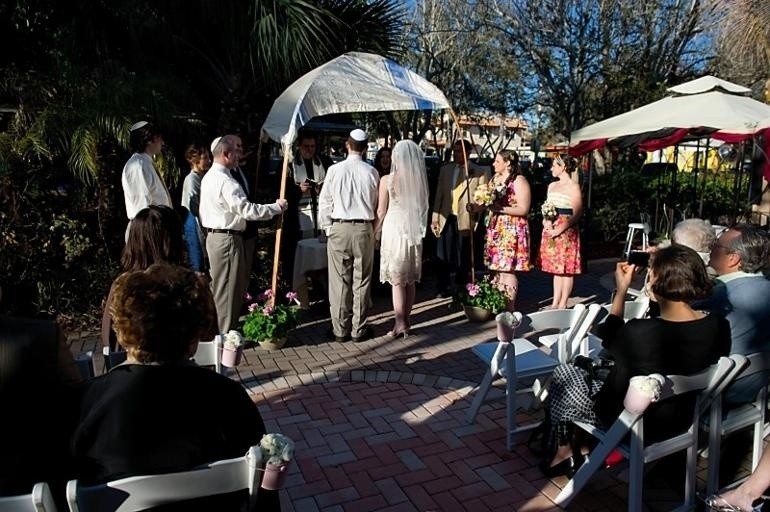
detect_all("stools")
[623,222,649,262]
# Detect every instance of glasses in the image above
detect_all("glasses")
[708,237,728,250]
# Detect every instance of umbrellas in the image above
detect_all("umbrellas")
[568,74,770,220]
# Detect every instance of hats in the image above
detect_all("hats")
[210,137,222,152]
[129,121,148,132]
[350,128,368,141]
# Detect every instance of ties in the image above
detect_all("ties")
[455,165,464,188]
[236,167,247,194]
[152,162,172,208]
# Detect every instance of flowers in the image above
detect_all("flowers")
[238,284,306,340]
[456,274,515,313]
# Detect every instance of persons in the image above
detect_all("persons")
[528,243,732,480]
[316,124,430,341]
[286,128,332,310]
[179,134,288,339]
[0,304,85,512]
[467,146,532,313]
[122,117,176,249]
[634,218,719,319]
[688,223,770,413]
[430,137,488,314]
[65,262,283,512]
[101,203,189,351]
[696,434,770,512]
[533,152,583,309]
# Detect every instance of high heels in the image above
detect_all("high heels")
[387,324,410,339]
[540,453,584,480]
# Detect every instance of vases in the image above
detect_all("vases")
[465,306,493,325]
[258,335,285,352]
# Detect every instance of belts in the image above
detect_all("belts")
[332,219,364,223]
[208,228,231,233]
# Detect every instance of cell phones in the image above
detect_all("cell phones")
[628,251,650,267]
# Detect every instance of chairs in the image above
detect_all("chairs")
[2,336,265,512]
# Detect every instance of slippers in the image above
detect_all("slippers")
[695,491,740,512]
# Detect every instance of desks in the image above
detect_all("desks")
[294,237,328,304]
